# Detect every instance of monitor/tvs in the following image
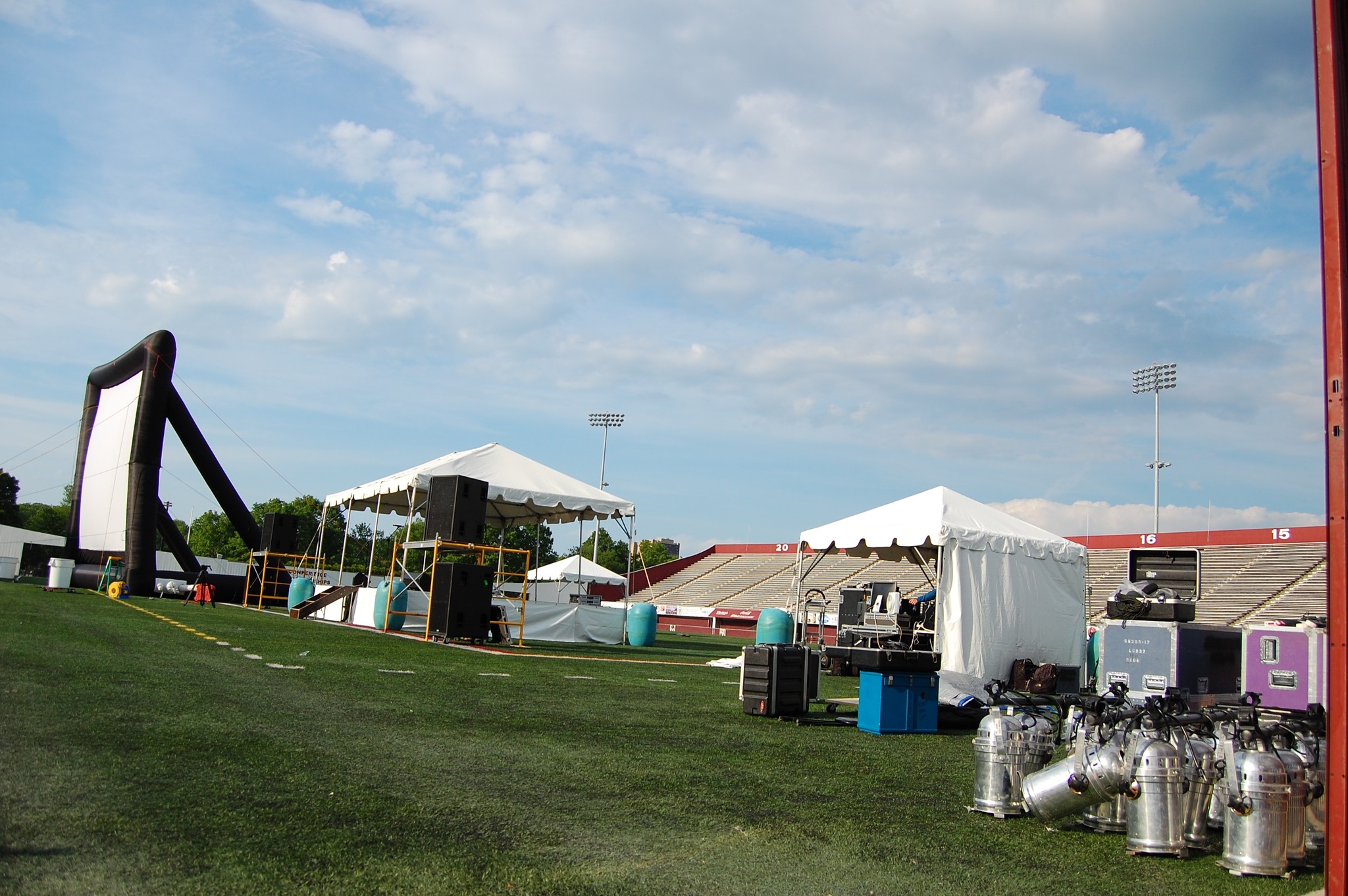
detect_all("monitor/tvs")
[870,582,897,614]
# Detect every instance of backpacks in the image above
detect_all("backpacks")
[1008,658,1060,696]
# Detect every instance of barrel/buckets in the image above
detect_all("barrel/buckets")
[48,557,75,589]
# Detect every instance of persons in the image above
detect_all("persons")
[909,556,943,605]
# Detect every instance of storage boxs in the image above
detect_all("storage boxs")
[1096,619,1243,701]
[1240,622,1328,713]
[863,612,911,629]
[1106,547,1203,622]
[856,669,939,735]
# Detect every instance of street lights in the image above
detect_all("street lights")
[588,410,625,565]
[1132,363,1177,534]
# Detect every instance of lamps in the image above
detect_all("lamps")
[970,706,1326,881]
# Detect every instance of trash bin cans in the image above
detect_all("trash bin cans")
[48,557,75,588]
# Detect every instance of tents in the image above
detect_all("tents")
[520,555,628,609]
[792,486,1087,707]
[315,443,635,644]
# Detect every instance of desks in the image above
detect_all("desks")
[840,624,934,649]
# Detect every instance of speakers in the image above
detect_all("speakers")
[428,562,494,638]
[242,565,277,607]
[260,513,299,555]
[743,643,812,716]
[886,591,899,614]
[422,476,489,545]
[352,571,371,588]
[403,572,431,593]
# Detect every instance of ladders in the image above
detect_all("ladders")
[98,556,122,594]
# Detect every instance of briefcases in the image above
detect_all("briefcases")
[1107,548,1201,622]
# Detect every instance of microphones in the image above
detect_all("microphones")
[393,525,404,528]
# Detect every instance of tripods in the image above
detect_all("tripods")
[184,565,216,608]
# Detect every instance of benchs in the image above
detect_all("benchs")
[616,552,937,613]
[1086,542,1327,627]
